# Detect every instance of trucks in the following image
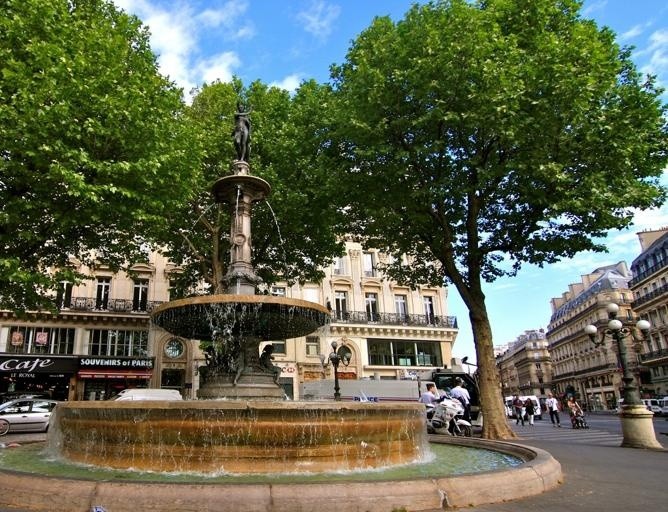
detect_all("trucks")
[297,369,481,426]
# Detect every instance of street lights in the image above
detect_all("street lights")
[319,341,352,399]
[584,303,651,404]
[494,354,506,404]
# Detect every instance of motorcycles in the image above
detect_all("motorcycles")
[425,396,472,436]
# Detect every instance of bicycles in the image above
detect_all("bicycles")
[0,418,9,436]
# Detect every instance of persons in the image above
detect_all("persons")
[199,344,282,386]
[545,393,560,427]
[418,384,440,419]
[232,101,252,161]
[524,398,534,425]
[513,394,524,425]
[567,396,584,429]
[449,378,471,422]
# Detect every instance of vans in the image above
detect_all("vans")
[505,396,542,419]
[103,388,184,401]
[616,397,668,417]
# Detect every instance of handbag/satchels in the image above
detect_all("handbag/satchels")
[549,407,553,415]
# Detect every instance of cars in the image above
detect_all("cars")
[0,399,58,433]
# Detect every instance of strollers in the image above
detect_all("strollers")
[570,408,588,429]
